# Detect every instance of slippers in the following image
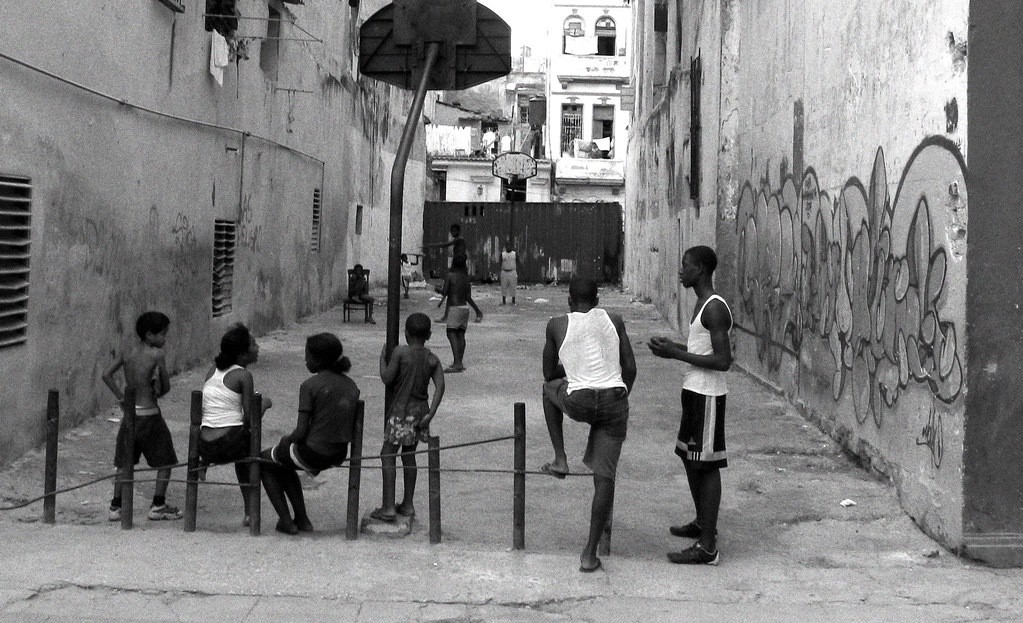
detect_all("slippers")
[396,503,417,516]
[371,507,397,522]
[541,463,566,481]
[578,557,601,573]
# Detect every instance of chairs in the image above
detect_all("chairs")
[342,269,370,325]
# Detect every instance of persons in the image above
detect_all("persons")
[419,224,483,323]
[646,245,733,566]
[101,311,183,522]
[499,241,521,306]
[370,313,445,521]
[258,332,360,535]
[399,254,420,299]
[540,278,637,573]
[434,253,471,373]
[192,321,272,526]
[350,264,376,324]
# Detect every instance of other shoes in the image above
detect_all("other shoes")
[275,519,296,535]
[367,317,376,324]
[434,318,445,322]
[475,313,483,323]
[294,519,315,532]
[353,297,361,303]
[444,365,466,373]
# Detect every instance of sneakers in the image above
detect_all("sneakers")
[671,521,719,541]
[668,543,720,566]
[108,505,121,522]
[147,501,183,520]
